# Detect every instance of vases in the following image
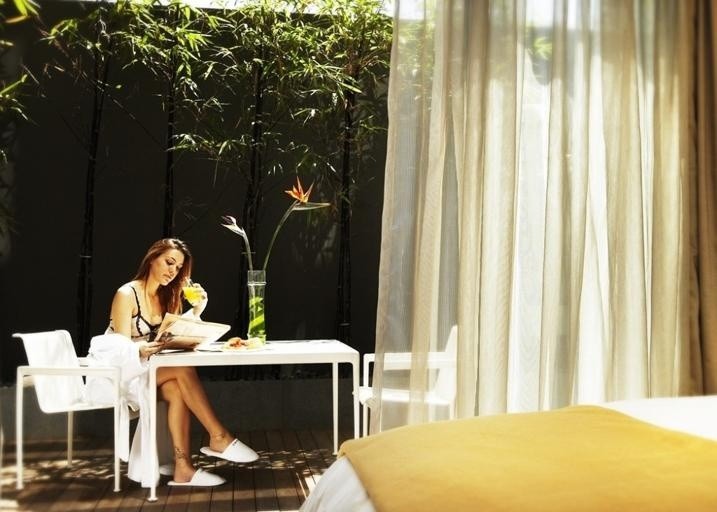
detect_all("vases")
[245,269,268,350]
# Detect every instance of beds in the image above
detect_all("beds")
[297,394,716,512]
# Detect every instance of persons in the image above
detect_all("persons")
[103,236,259,488]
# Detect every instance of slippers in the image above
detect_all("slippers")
[167,467,228,487]
[199,437,260,464]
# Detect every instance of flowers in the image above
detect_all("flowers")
[217,172,333,335]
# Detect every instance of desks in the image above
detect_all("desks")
[148,336,361,503]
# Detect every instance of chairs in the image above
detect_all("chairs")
[353,323,459,436]
[9,328,141,493]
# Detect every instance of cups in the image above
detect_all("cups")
[179,276,202,306]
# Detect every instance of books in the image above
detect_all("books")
[152,312,232,351]
